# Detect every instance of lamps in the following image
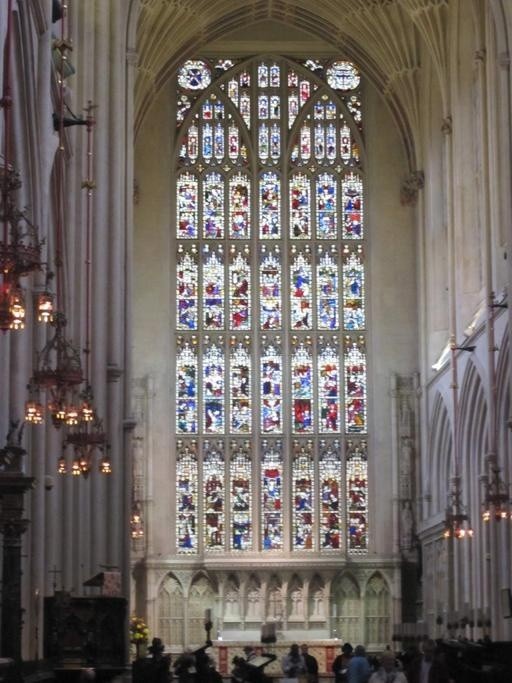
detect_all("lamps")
[130,500,148,541]
[57,123,113,480]
[0,0,59,335]
[477,308,509,524]
[439,352,476,540]
[23,117,95,431]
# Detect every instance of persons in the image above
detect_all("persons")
[242,638,454,683]
